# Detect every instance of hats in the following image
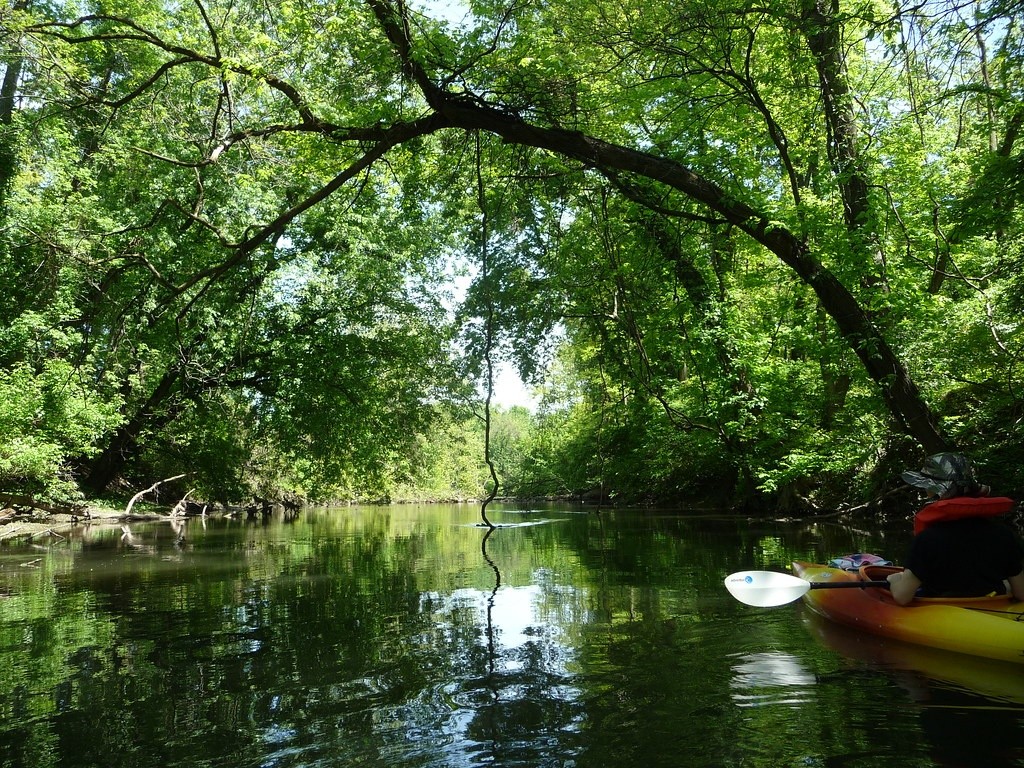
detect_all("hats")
[899,451,990,499]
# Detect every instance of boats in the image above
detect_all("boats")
[790,556,1024,663]
[797,603,1024,704]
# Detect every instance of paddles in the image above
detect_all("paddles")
[718,567,893,614]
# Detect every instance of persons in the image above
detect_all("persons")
[886,452,1023,606]
